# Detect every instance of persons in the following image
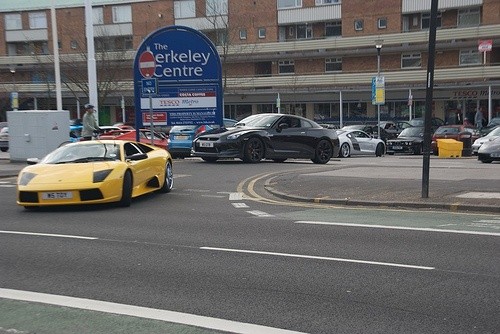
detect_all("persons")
[475,107,482,128]
[81,104,104,141]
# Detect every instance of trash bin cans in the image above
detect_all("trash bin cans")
[459,131,473,156]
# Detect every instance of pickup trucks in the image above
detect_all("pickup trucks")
[431,126,480,155]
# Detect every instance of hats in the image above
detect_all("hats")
[85,105,93,110]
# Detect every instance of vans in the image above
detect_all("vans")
[410,117,447,128]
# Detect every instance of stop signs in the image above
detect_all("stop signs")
[138,51,156,79]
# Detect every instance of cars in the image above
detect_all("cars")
[472,127,500,154]
[478,137,500,163]
[380,121,411,133]
[337,125,391,140]
[70,125,170,142]
[387,127,435,155]
[190,113,340,164]
[336,130,386,157]
[0,126,8,151]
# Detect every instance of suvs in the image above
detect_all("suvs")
[168,119,240,153]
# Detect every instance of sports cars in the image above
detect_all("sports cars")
[16,140,173,211]
